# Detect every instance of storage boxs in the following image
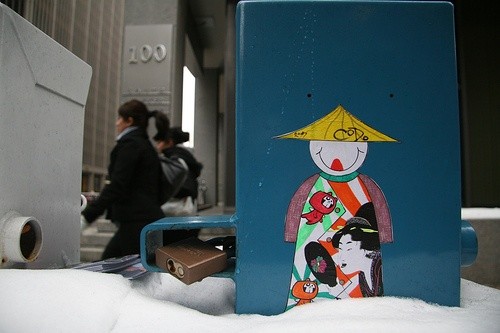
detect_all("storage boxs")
[141,0,478,315]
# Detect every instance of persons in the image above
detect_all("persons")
[81,100,169,260]
[154,127,203,216]
